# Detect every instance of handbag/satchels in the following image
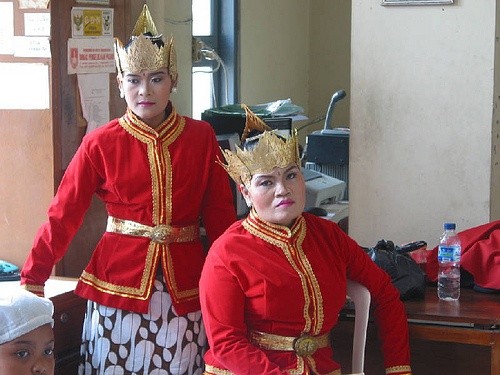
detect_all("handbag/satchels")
[344,239,426,318]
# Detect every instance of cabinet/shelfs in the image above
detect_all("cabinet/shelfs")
[19,276,88,375]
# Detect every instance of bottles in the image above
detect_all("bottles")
[438,223,461,301]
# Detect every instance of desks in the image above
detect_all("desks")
[336,288,500,375]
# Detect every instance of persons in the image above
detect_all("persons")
[20,4,234,375]
[0,288,55,375]
[199,104,412,375]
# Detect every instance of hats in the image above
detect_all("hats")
[0,289,54,345]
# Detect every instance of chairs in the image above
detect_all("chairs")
[332,276,371,373]
